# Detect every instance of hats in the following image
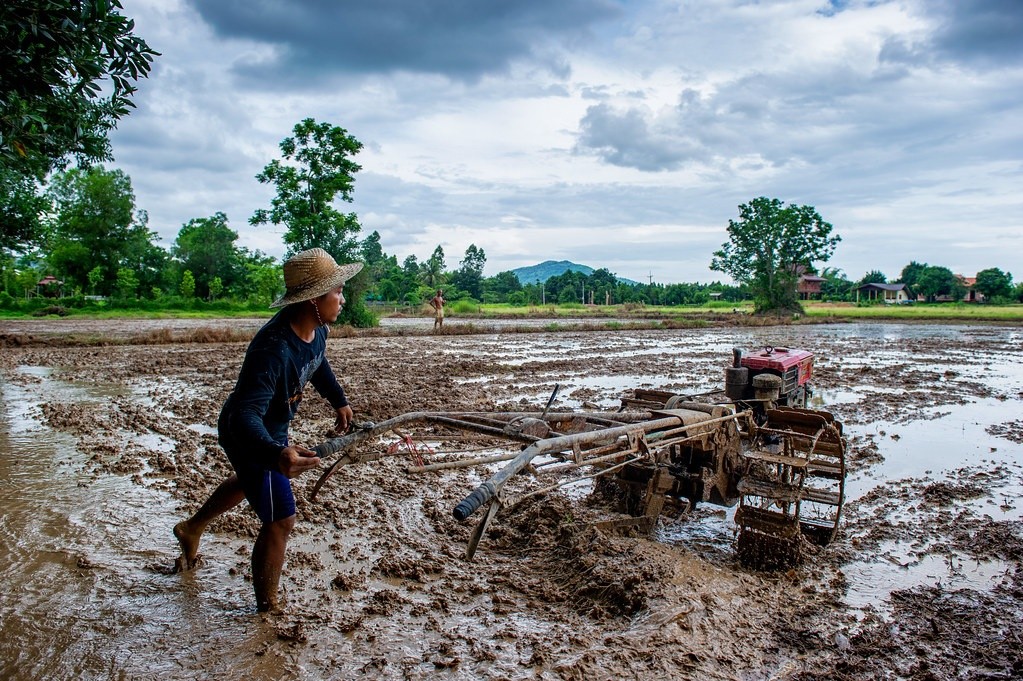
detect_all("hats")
[268,247,365,310]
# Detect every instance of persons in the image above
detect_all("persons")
[429,289,446,328]
[173,248,364,614]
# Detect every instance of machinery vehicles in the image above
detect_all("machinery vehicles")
[299,343,847,567]
